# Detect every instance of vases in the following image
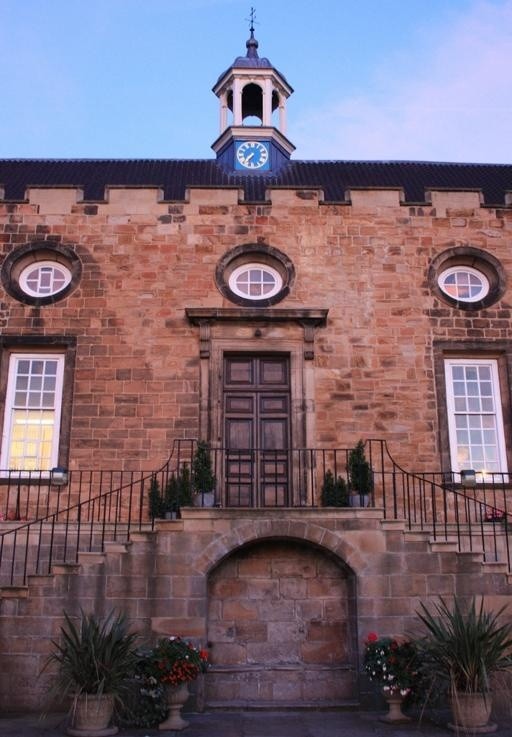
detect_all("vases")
[157,680,191,731]
[377,687,413,725]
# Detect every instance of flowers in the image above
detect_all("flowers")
[142,633,213,689]
[357,632,428,697]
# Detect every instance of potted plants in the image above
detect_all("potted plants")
[344,438,376,507]
[29,600,153,737]
[147,462,194,523]
[190,439,218,508]
[403,589,512,734]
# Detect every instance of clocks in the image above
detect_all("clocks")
[236,140,269,170]
